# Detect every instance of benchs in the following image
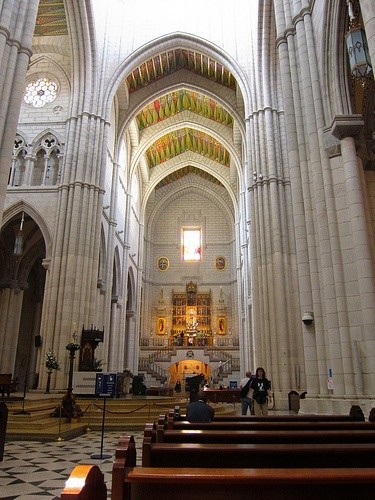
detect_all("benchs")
[60,403,375,500]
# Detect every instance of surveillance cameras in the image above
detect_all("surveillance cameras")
[302,312,315,325]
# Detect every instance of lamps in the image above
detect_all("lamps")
[14,212,24,263]
[301,312,314,326]
[344,0,372,78]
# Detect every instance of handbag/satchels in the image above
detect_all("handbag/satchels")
[240,384,250,398]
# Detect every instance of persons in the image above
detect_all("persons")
[197,331,202,337]
[250,367,270,416]
[186,392,214,421]
[240,372,254,415]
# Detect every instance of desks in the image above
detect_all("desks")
[147,387,173,396]
[203,389,243,402]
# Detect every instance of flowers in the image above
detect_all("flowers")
[43,348,62,372]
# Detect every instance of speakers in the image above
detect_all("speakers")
[35,335,41,347]
